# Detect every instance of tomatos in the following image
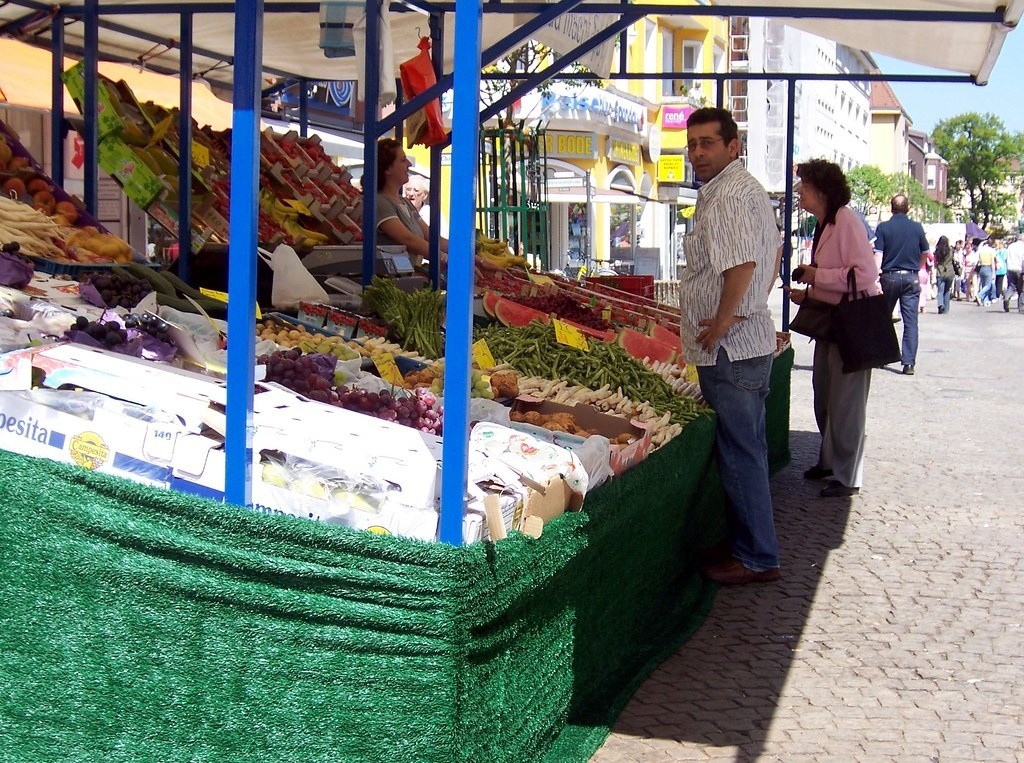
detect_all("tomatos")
[281,138,365,242]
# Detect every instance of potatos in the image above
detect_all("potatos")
[490,371,639,452]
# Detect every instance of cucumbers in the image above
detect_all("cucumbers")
[104,259,229,317]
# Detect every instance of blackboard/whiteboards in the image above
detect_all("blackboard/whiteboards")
[633,247,660,280]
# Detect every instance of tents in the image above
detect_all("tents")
[926,223,990,251]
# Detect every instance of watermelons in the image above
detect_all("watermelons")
[481,292,689,369]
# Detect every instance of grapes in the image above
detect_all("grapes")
[0,242,176,351]
[251,342,494,437]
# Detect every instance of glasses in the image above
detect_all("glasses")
[684,137,723,152]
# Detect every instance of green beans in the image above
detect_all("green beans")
[472,315,715,425]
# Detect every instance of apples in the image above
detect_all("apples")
[0,137,78,229]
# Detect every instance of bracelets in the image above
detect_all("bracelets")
[479,259,485,265]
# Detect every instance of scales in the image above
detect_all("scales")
[301,245,416,279]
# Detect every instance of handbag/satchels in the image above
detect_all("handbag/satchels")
[788,282,834,349]
[952,261,961,276]
[828,269,904,375]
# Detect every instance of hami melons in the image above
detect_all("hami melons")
[100,86,182,194]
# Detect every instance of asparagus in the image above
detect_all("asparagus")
[358,274,448,357]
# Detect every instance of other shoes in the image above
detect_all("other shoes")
[938,306,945,315]
[819,480,860,497]
[804,463,834,480]
[956,298,962,301]
[991,298,999,304]
[902,364,916,376]
[970,295,973,298]
[975,295,982,306]
[1003,299,1010,312]
[699,558,780,585]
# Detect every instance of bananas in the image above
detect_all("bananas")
[257,187,329,246]
[473,226,532,269]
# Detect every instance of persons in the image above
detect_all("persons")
[778,159,1024,498]
[677,108,785,584]
[375,138,513,283]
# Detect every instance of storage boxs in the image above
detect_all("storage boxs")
[0,270,792,547]
[139,102,364,255]
[98,136,208,210]
[62,59,153,148]
